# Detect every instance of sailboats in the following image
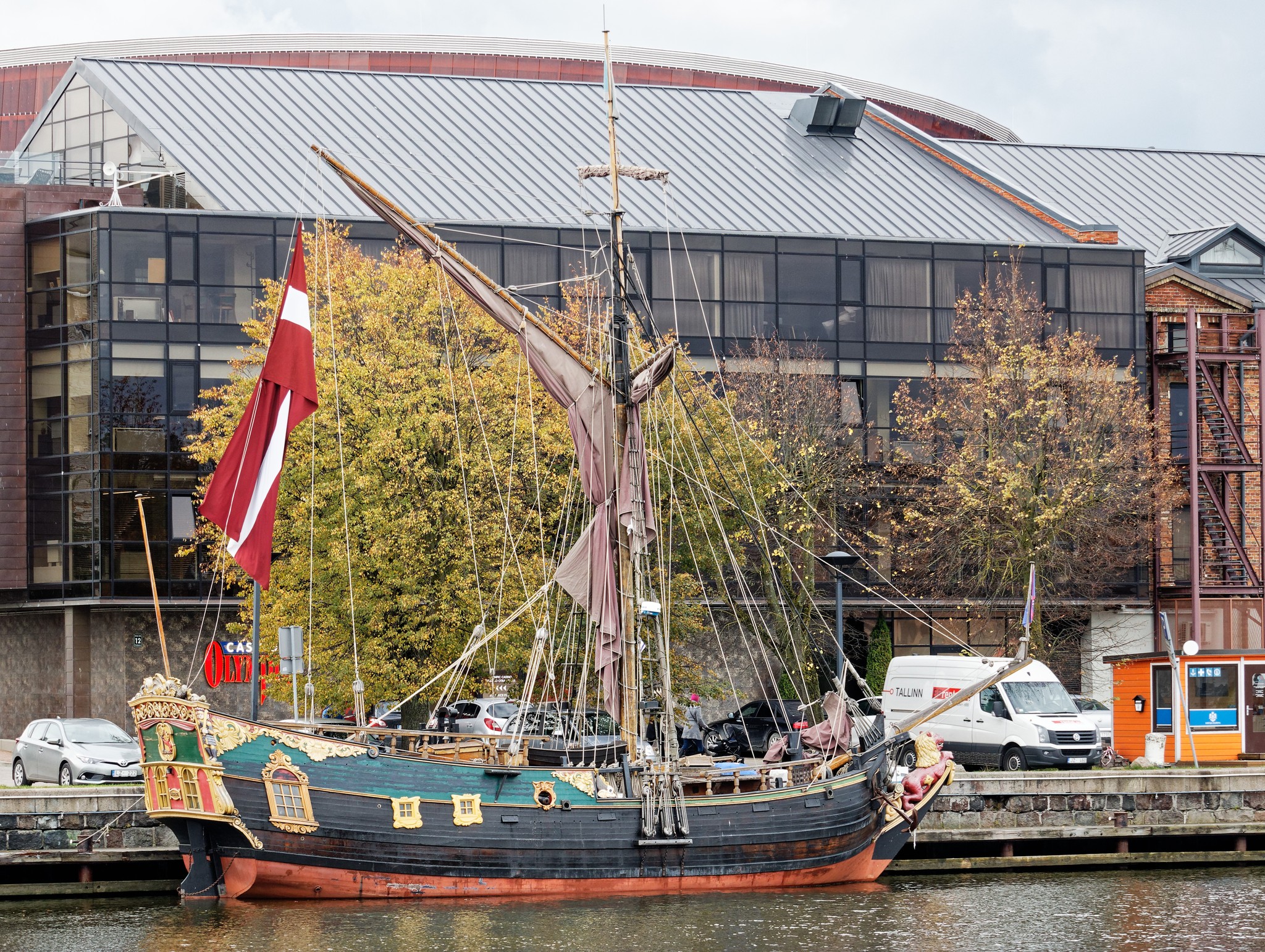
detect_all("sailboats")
[123,20,1039,909]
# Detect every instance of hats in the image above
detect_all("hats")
[691,693,699,703]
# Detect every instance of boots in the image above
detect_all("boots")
[678,748,686,759]
[697,750,704,756]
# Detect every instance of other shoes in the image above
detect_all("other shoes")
[662,757,667,762]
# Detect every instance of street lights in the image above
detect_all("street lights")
[813,551,863,695]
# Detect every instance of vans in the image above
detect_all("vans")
[881,655,1102,772]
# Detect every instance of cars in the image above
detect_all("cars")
[844,695,884,749]
[498,709,657,764]
[1067,694,1112,744]
[702,698,829,760]
[435,698,534,744]
[10,716,143,786]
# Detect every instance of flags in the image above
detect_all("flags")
[1022,563,1036,629]
[199,219,320,592]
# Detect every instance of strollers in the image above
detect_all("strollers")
[702,723,750,764]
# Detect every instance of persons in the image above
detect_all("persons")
[646,698,668,760]
[677,694,711,758]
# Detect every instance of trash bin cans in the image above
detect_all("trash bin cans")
[1145,732,1167,766]
